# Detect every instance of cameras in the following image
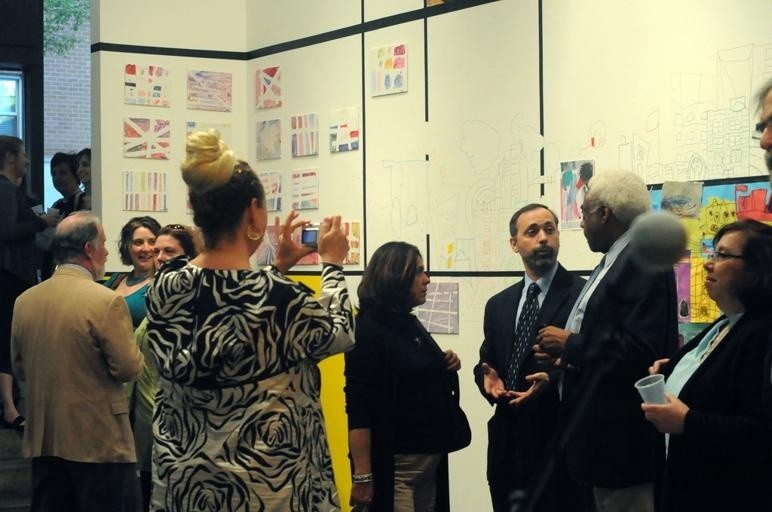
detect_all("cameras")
[301,224,322,248]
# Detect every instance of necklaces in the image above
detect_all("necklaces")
[126,269,155,286]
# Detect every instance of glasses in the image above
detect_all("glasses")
[706,252,739,261]
[164,224,185,229]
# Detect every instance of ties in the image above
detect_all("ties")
[504,284,541,392]
[556,257,604,402]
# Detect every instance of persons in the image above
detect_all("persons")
[753,79,771,173]
[471,202,590,511]
[3,137,91,430]
[10,210,144,510]
[533,168,674,511]
[123,224,196,509]
[102,216,161,332]
[342,238,469,510]
[638,220,771,511]
[137,126,357,510]
[571,162,593,225]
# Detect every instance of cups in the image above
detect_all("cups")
[633,373,668,406]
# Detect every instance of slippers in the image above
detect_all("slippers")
[1,413,27,430]
